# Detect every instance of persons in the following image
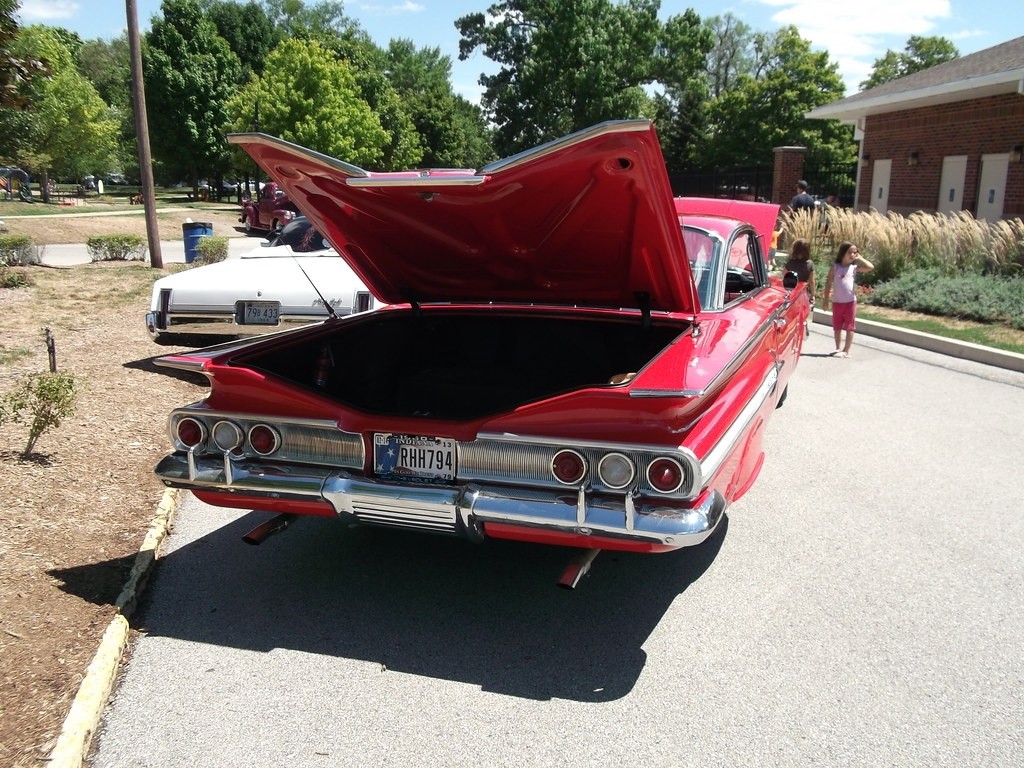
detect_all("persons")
[784,179,816,228]
[766,226,784,272]
[782,238,817,336]
[822,241,875,359]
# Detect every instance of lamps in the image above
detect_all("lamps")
[1009,144,1024,162]
[908,152,918,166]
[860,155,870,167]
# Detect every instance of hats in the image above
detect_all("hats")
[795,179,807,188]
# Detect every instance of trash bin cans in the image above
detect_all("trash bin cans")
[182,220,213,263]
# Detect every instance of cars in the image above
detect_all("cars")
[152,118,807,592]
[145,214,390,349]
[237,182,305,234]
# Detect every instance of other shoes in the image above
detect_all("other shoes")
[831,349,848,358]
